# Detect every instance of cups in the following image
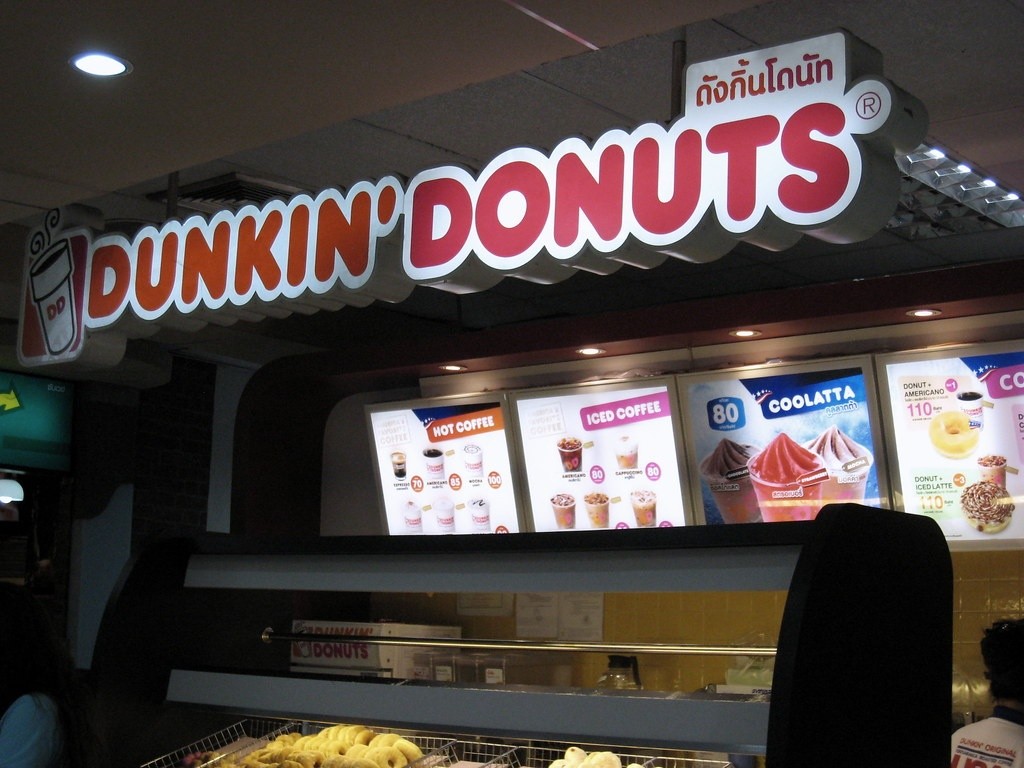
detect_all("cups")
[464,449,483,478]
[556,442,582,473]
[550,497,576,529]
[612,441,639,469]
[404,507,423,531]
[748,450,823,522]
[423,449,445,480]
[977,459,1007,491]
[390,453,406,481]
[698,451,763,524]
[631,500,657,527]
[817,444,874,506]
[956,392,984,431]
[584,498,610,528]
[470,505,491,532]
[434,504,456,533]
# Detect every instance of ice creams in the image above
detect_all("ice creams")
[699,424,874,522]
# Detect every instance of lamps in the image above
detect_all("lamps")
[985,193,1019,203]
[961,179,996,191]
[906,146,944,163]
[935,164,972,178]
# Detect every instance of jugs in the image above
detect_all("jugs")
[597,655,645,691]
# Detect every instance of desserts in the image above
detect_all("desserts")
[961,479,1015,533]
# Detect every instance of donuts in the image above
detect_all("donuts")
[177,723,426,768]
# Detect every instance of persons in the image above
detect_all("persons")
[949,619,1024,768]
[0,582,111,768]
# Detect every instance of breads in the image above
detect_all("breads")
[548,747,643,768]
